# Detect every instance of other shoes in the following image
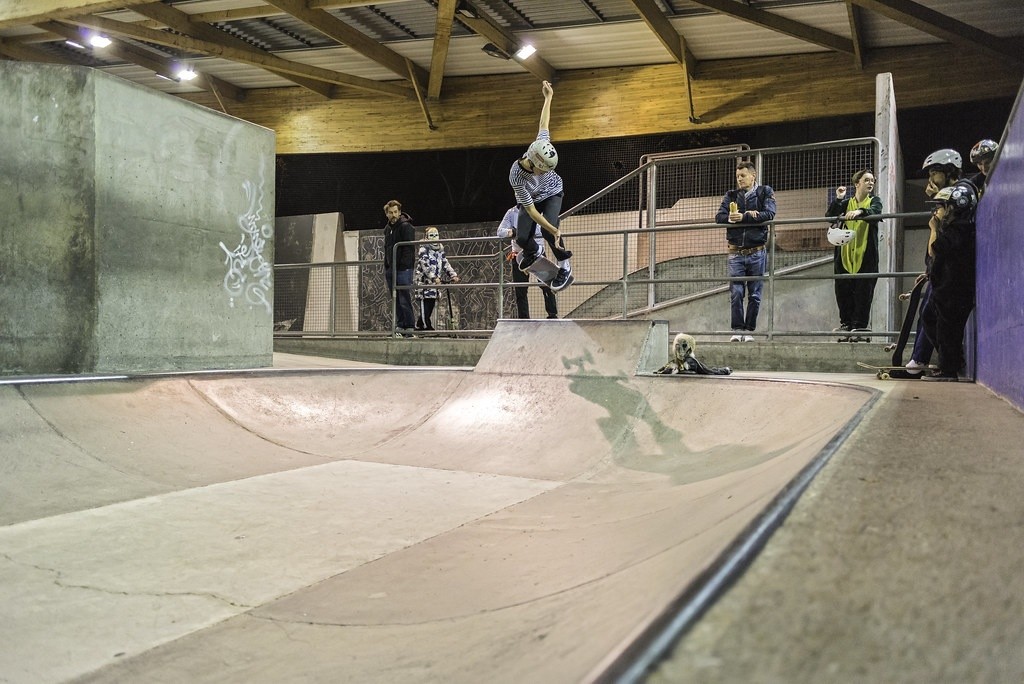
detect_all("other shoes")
[834,323,851,332]
[387,327,406,337]
[418,326,424,330]
[888,366,925,379]
[406,328,414,338]
[426,325,435,331]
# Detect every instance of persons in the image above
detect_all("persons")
[384,200,416,337]
[414,226,458,337]
[715,162,777,342]
[654,334,730,375]
[969,140,999,188]
[509,81,573,291]
[497,205,558,319]
[824,169,884,342]
[888,150,980,381]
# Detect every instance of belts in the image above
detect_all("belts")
[729,246,765,255]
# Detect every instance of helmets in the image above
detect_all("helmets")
[922,148,963,170]
[925,185,971,210]
[826,226,855,246]
[969,140,998,162]
[526,140,558,171]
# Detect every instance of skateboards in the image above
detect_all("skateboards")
[516,249,575,293]
[856,363,963,380]
[883,275,929,367]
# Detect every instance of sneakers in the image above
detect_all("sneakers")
[921,370,959,382]
[550,267,572,290]
[730,334,742,341]
[519,245,544,271]
[743,334,755,342]
